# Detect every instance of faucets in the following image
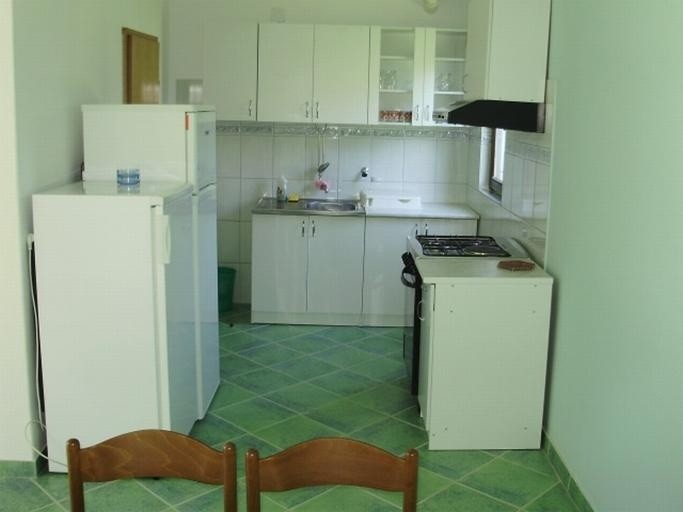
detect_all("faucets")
[320,183,329,194]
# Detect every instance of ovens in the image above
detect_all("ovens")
[402,251,423,399]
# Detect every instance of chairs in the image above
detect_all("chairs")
[67,429,236,512]
[245,438,418,512]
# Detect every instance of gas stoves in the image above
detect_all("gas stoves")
[417,233,507,259]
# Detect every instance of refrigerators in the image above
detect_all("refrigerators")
[32,181,196,472]
[81,104,220,419]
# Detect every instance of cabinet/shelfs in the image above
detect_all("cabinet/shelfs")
[256,25,368,125]
[251,214,364,326]
[461,1,551,103]
[204,22,258,122]
[362,216,477,327]
[416,259,554,450]
[369,27,465,128]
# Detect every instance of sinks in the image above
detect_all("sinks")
[304,199,360,212]
